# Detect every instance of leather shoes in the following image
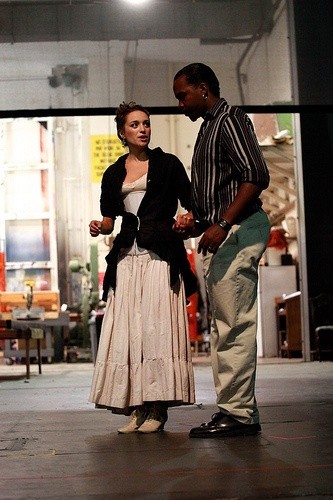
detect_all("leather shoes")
[188,412,222,438]
[201,413,261,439]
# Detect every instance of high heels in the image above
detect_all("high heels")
[117,409,145,434]
[137,412,168,433]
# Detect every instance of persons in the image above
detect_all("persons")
[89,101,196,435]
[171,63,270,438]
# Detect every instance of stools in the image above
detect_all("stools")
[0,327,45,379]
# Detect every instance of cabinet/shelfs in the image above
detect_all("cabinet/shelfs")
[273,291,302,359]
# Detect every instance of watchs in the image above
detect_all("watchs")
[218,218,231,231]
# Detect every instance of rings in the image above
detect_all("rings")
[208,246,212,250]
